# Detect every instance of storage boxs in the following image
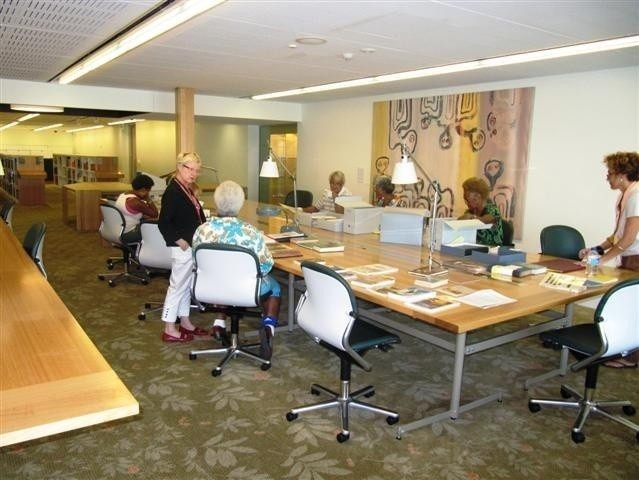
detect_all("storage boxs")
[334,195,493,252]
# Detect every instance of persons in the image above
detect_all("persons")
[304,169,351,213]
[157,154,207,342]
[114,175,158,244]
[194,182,280,361]
[375,178,407,207]
[457,178,504,246]
[579,152,638,370]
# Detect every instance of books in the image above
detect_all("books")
[264,230,462,315]
[488,258,586,277]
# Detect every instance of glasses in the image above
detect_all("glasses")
[184,164,200,173]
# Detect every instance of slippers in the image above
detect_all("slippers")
[603,359,638,369]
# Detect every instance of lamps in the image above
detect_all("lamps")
[48,0,228,85]
[390,143,448,278]
[249,34,638,101]
[259,147,300,234]
[0,113,145,133]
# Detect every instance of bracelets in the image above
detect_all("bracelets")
[597,246,604,255]
[148,199,153,205]
[616,244,625,252]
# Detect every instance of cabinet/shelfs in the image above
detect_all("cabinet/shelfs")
[0,154,47,206]
[53,154,125,186]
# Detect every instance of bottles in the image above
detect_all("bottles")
[586,248,601,277]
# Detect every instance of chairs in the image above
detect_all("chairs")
[188,243,272,376]
[539,223,586,351]
[23,220,48,279]
[0,187,18,231]
[98,197,149,287]
[527,279,639,444]
[501,219,515,248]
[286,262,402,442]
[136,222,205,324]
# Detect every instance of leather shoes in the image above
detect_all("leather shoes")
[213,326,227,339]
[179,324,209,336]
[162,331,194,341]
[259,326,272,360]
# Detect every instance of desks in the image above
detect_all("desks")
[201,193,639,436]
[61,182,247,234]
[0,217,140,447]
